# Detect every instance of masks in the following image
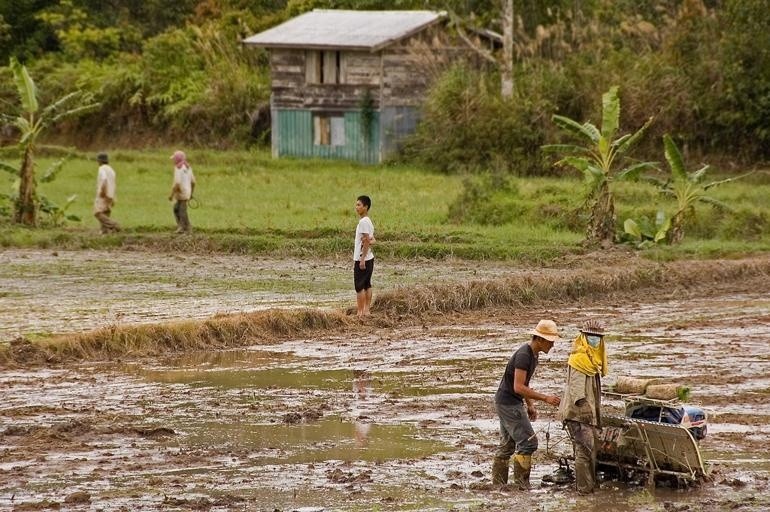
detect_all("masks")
[587,336,600,347]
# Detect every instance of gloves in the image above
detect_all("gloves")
[576,399,595,425]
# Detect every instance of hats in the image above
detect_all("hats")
[580,319,609,336]
[525,320,564,343]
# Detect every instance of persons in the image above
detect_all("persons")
[557,318,607,495]
[93,152,122,235]
[352,194,377,317]
[168,149,197,234]
[492,317,562,488]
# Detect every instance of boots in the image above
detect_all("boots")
[492,456,509,492]
[513,452,532,491]
